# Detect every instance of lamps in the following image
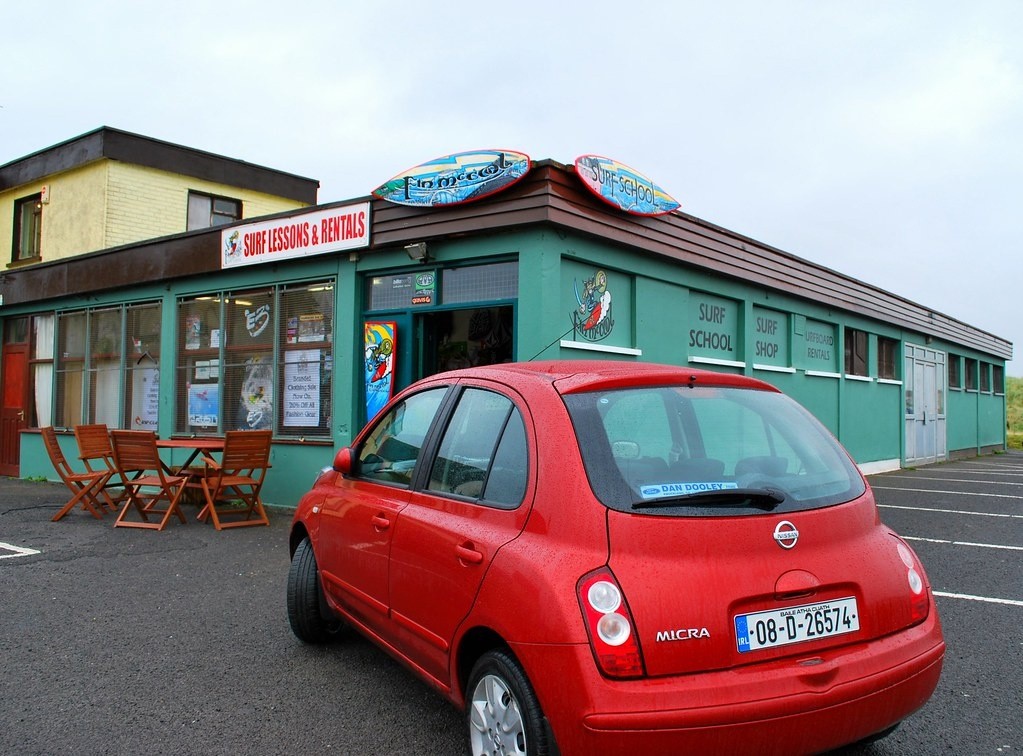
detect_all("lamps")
[405,241,435,264]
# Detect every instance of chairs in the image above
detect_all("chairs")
[73,424,146,512]
[611,455,791,491]
[40,425,111,522]
[444,434,508,501]
[196,429,275,531]
[110,428,190,531]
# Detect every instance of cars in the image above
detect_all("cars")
[287,359,951,756]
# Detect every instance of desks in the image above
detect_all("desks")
[151,440,227,485]
